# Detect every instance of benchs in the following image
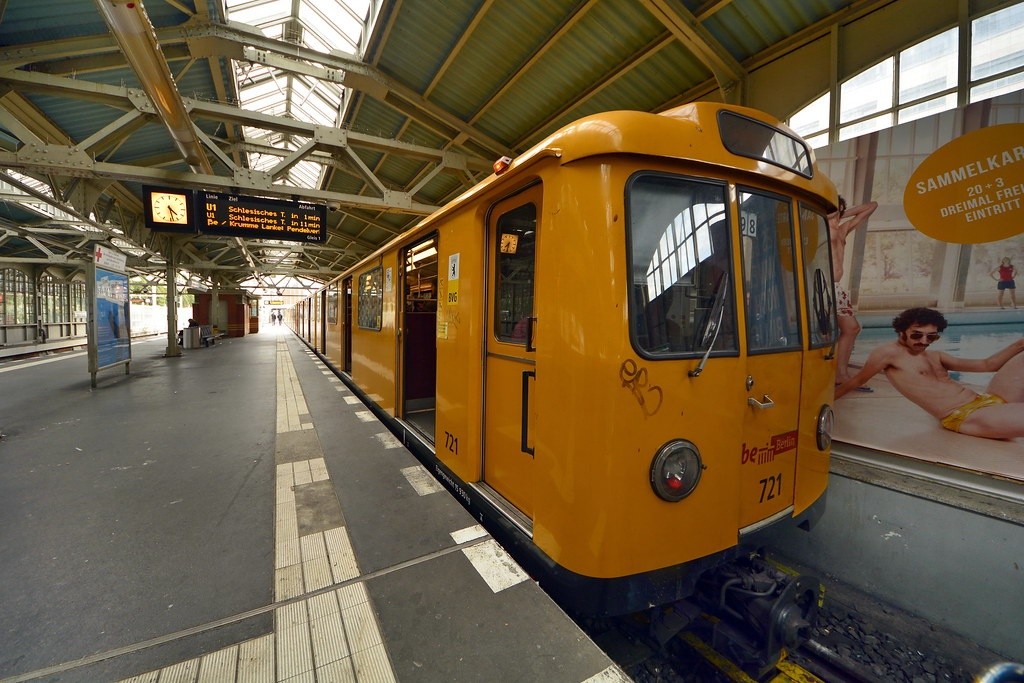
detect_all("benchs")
[198,325,224,347]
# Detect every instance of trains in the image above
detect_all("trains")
[287,102,844,674]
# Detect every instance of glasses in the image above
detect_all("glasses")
[910,334,940,340]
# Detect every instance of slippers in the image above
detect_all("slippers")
[835,383,873,393]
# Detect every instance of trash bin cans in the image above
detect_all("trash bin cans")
[183,328,200,349]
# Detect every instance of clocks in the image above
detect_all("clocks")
[142,185,194,227]
[500,231,522,255]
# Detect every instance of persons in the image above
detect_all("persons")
[827,195,877,392]
[278,313,282,326]
[178,319,198,345]
[838,306,1024,438]
[406,279,437,312]
[989,257,1018,308]
[510,302,532,339]
[271,312,276,325]
